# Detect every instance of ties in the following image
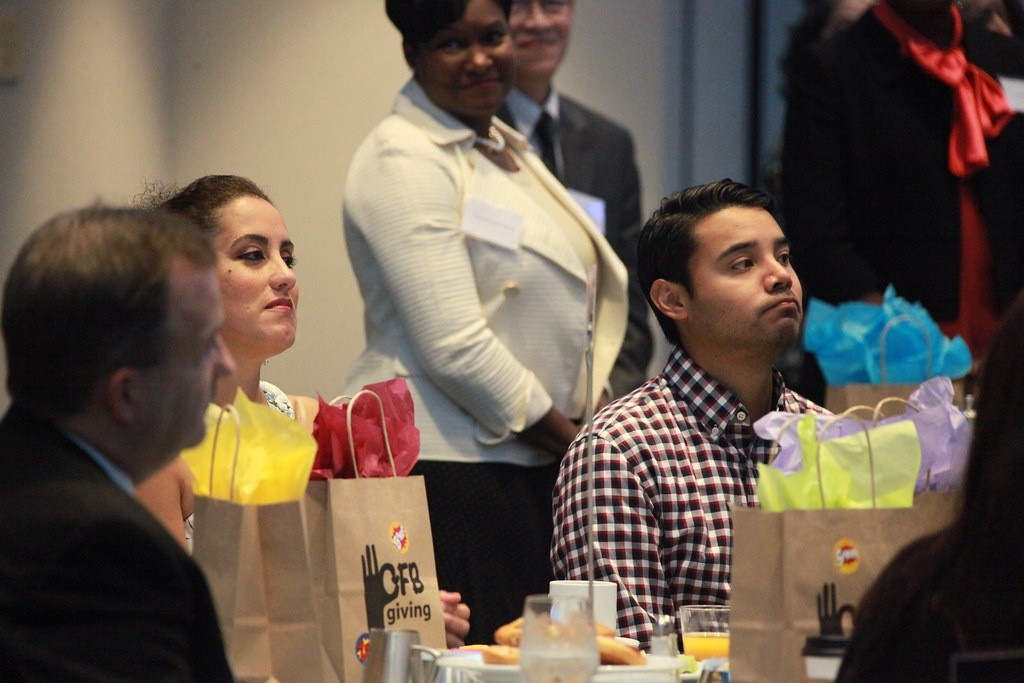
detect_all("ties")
[534,109,558,180]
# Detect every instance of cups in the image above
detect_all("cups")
[518,595,600,683]
[360,628,442,683]
[679,603,733,662]
[801,636,851,680]
[547,579,616,634]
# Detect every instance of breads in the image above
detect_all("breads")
[481,615,646,666]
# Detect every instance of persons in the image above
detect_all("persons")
[779,1,1016,368]
[341,0,628,647]
[833,306,1024,683]
[135,175,472,649]
[550,179,845,651]
[491,0,655,415]
[0,205,236,683]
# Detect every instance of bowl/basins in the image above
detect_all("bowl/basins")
[435,652,687,683]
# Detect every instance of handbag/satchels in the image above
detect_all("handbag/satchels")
[729,396,965,683]
[191,400,342,683]
[826,315,965,419]
[302,389,447,683]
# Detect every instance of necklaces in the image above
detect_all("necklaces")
[475,125,507,154]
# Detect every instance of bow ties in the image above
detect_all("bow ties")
[872,1,1017,178]
